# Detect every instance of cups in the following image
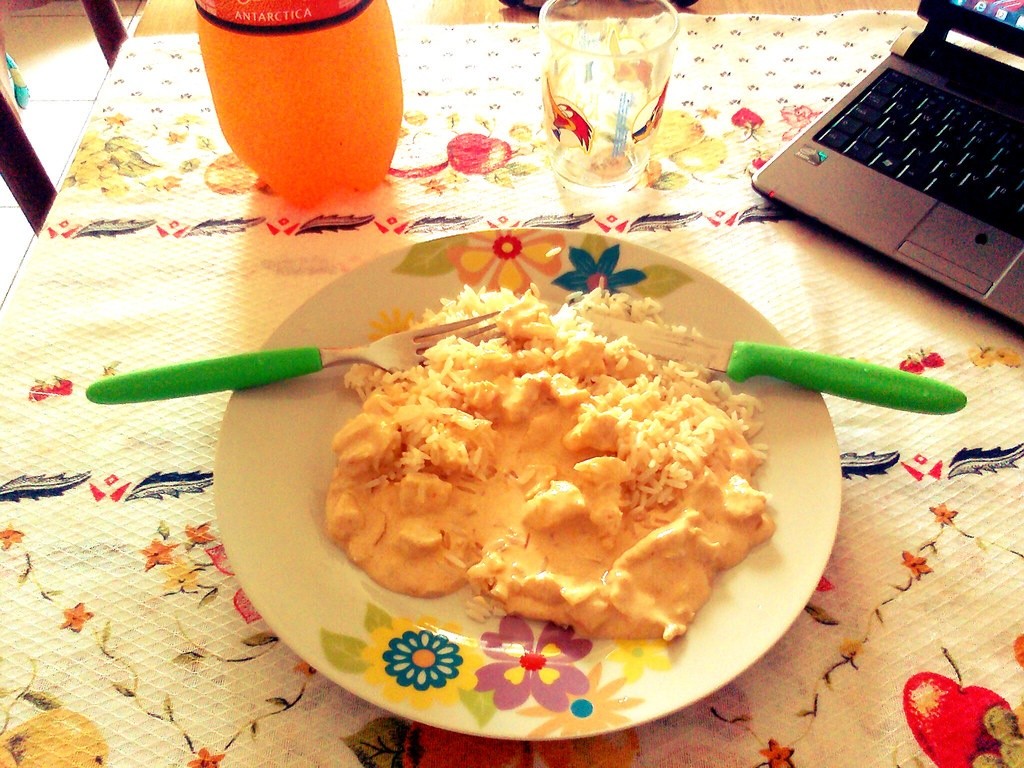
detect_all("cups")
[539,1,679,188]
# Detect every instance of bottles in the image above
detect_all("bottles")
[194,1,403,211]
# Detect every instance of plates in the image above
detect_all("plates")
[214,227,840,740]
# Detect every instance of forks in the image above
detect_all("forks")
[87,311,504,404]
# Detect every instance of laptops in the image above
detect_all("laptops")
[746,1,1024,334]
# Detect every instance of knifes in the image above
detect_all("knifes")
[580,310,967,415]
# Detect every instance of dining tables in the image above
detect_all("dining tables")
[1,0,1023,768]
